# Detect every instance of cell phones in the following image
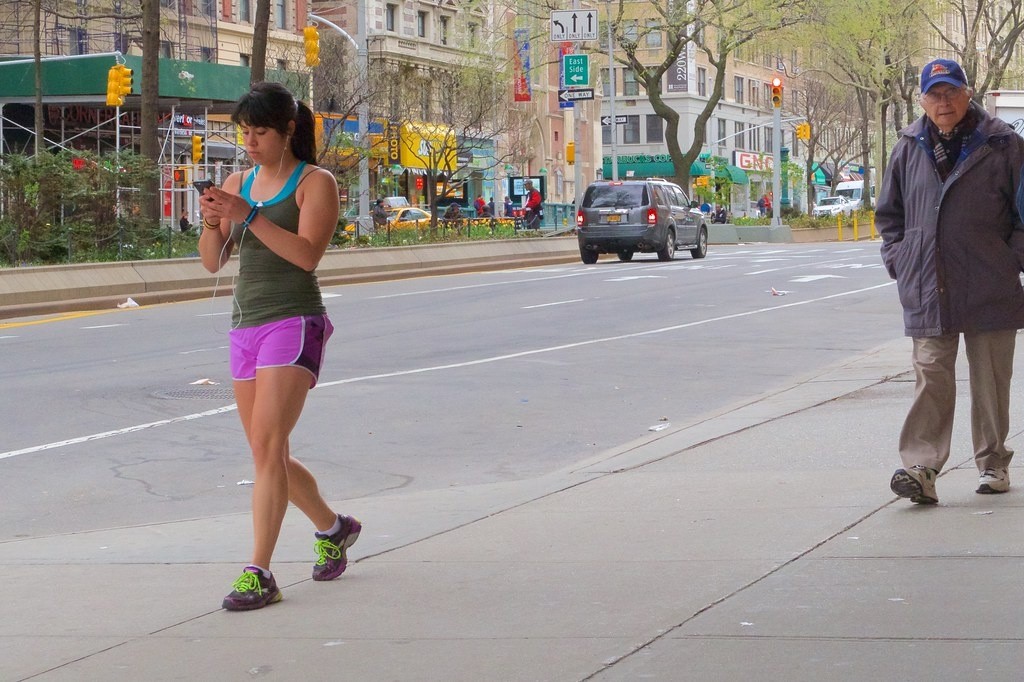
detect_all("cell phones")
[193,180,217,202]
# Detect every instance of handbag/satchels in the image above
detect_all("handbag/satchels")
[534,205,544,220]
[474,201,479,211]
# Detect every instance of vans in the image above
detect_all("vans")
[834,179,875,209]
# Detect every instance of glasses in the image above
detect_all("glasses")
[921,85,964,104]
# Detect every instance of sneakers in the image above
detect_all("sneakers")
[890,463,938,504]
[976,465,1011,493]
[311,512,362,581]
[222,567,283,611]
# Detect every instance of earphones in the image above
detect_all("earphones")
[285,129,292,140]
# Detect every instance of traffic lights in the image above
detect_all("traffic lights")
[192,135,205,164]
[772,77,782,107]
[797,124,804,138]
[174,169,188,183]
[119,65,133,95]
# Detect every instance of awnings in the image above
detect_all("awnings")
[808,162,866,187]
[601,163,750,185]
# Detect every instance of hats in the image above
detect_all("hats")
[920,59,969,94]
[523,180,532,183]
[450,203,461,207]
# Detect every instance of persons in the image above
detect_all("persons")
[873,59,1023,506]
[710,203,726,224]
[503,196,513,217]
[373,199,390,228]
[756,195,770,215]
[487,197,495,215]
[444,203,495,229]
[197,83,363,611]
[180,211,194,234]
[522,180,542,229]
[474,195,485,218]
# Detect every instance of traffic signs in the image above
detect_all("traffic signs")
[601,116,628,125]
[563,54,589,87]
[549,9,598,41]
[558,88,595,102]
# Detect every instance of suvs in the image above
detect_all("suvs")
[578,180,708,264]
[811,195,851,218]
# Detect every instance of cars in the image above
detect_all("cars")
[344,200,377,217]
[710,208,726,224]
[340,207,442,240]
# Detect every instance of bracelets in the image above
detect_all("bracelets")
[241,205,259,228]
[203,218,221,230]
